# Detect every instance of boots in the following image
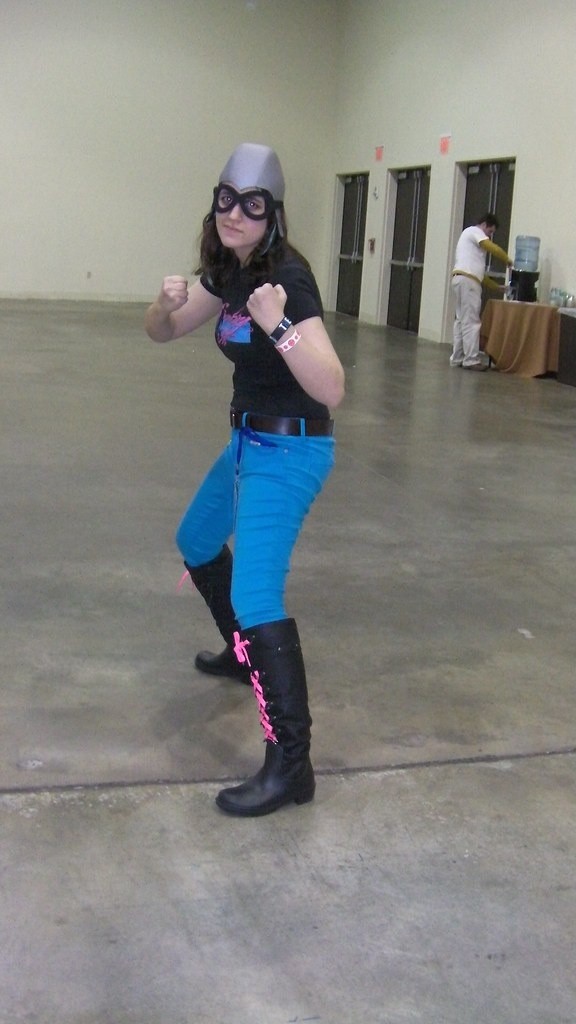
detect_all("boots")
[182,543,260,686]
[214,616,317,816]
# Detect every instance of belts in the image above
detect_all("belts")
[228,407,336,437]
[452,273,462,277]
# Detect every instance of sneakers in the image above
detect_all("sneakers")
[454,364,490,372]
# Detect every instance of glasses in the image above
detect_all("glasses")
[211,184,281,222]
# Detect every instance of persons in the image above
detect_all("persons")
[448,213,512,370]
[143,145,345,822]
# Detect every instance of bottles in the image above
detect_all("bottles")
[512,234,540,273]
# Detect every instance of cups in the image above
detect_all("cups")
[505,286,513,296]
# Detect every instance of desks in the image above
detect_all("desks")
[557,308,576,386]
[480,298,562,380]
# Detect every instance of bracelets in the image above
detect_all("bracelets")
[267,315,301,354]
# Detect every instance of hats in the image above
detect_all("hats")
[216,142,285,203]
